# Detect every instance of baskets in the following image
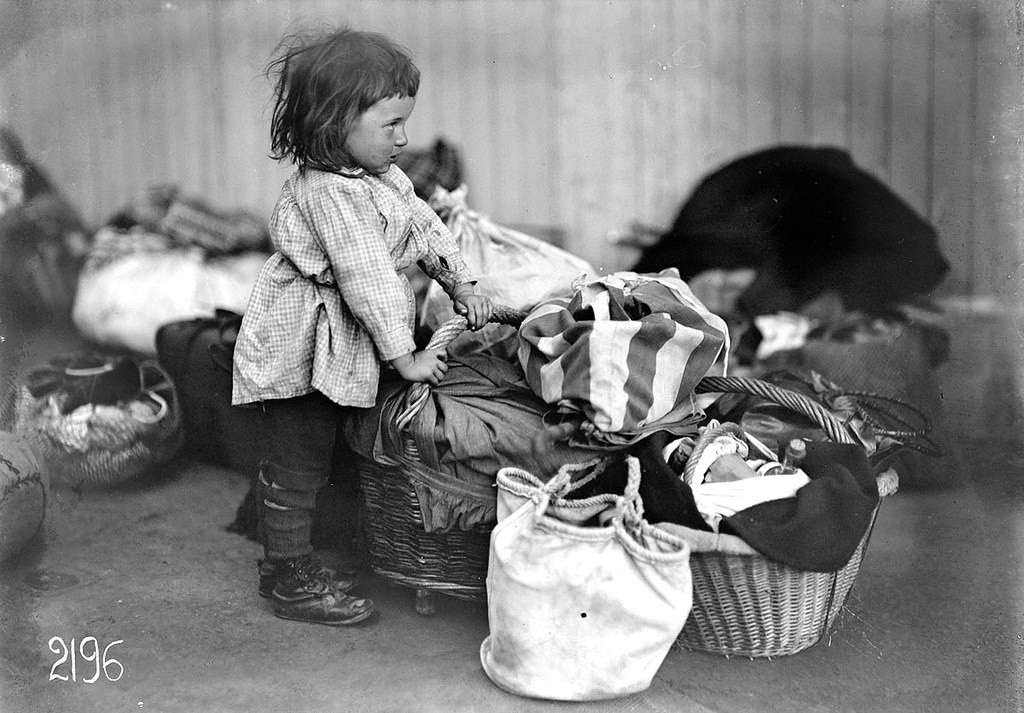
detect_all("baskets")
[357,305,532,615]
[674,375,900,657]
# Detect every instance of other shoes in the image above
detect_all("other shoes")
[272,554,374,625]
[257,555,359,601]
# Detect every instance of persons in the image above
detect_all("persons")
[228,23,493,629]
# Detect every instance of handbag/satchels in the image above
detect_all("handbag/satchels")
[478,456,694,701]
[518,265,730,432]
[804,306,968,491]
[156,307,259,477]
